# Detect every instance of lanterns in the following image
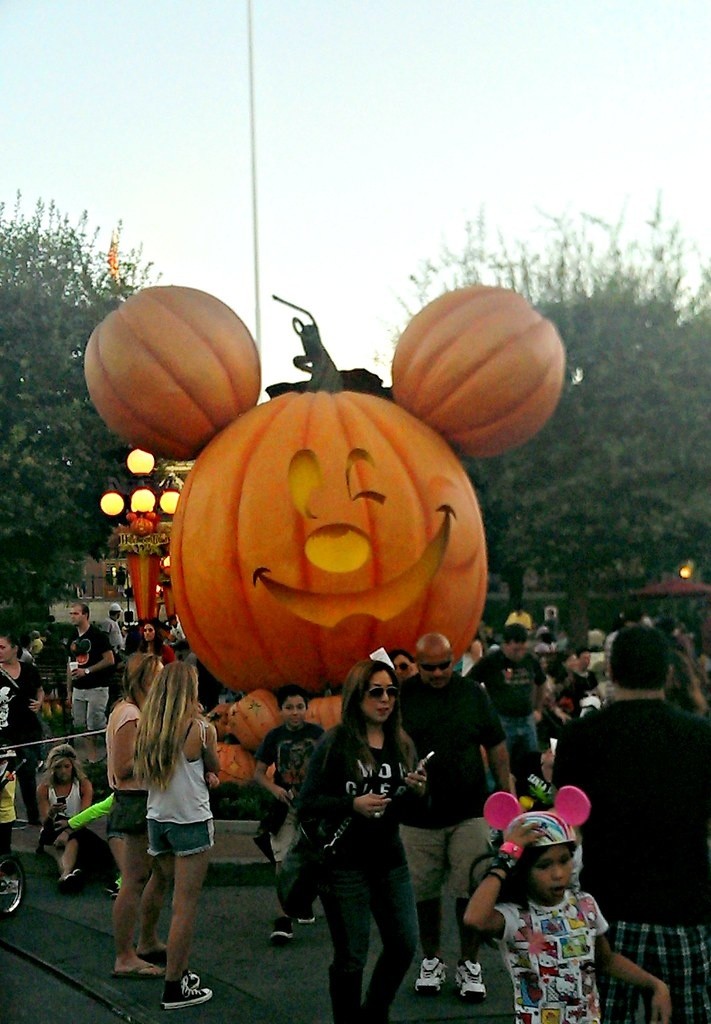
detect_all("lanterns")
[168,297,487,694]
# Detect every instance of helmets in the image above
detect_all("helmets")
[482,785,593,849]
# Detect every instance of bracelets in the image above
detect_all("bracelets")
[488,842,524,875]
[483,871,506,883]
[64,830,69,836]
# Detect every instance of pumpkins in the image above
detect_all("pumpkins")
[85,286,260,460]
[392,288,565,459]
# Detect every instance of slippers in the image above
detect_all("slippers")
[109,949,167,980]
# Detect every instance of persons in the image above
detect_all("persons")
[460,605,708,782]
[106,650,167,979]
[115,566,127,597]
[297,659,428,1024]
[0,604,187,900]
[400,634,513,1003]
[550,625,711,1024]
[464,786,675,1024]
[134,658,223,1009]
[252,684,326,942]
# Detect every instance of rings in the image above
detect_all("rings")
[374,812,381,818]
[424,777,427,781]
[418,782,422,787]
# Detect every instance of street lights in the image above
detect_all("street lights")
[101,447,180,620]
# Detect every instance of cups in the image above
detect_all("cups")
[69,662,79,680]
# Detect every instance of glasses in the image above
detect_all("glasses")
[368,686,399,698]
[418,659,451,672]
[393,662,409,671]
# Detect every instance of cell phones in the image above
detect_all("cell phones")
[411,752,434,780]
[57,796,66,806]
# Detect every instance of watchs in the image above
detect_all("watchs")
[85,668,89,675]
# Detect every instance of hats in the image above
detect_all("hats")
[110,603,122,611]
[165,614,177,622]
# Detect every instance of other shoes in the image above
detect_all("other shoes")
[11,820,28,830]
[270,917,294,941]
[58,869,83,894]
[297,902,316,924]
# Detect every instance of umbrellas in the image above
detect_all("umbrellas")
[628,579,711,598]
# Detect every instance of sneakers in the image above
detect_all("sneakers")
[161,970,212,1009]
[414,955,448,992]
[456,960,487,999]
